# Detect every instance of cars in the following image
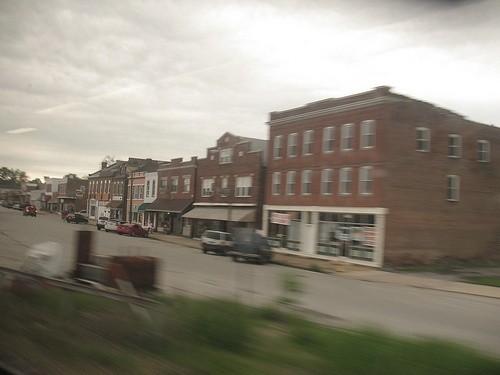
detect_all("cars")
[65,213,89,223]
[24,205,38,216]
[0,198,28,212]
[117,222,149,237]
[105,220,128,233]
[75,210,89,218]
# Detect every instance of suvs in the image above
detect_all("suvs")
[97,216,111,230]
[232,233,273,264]
[201,230,237,256]
